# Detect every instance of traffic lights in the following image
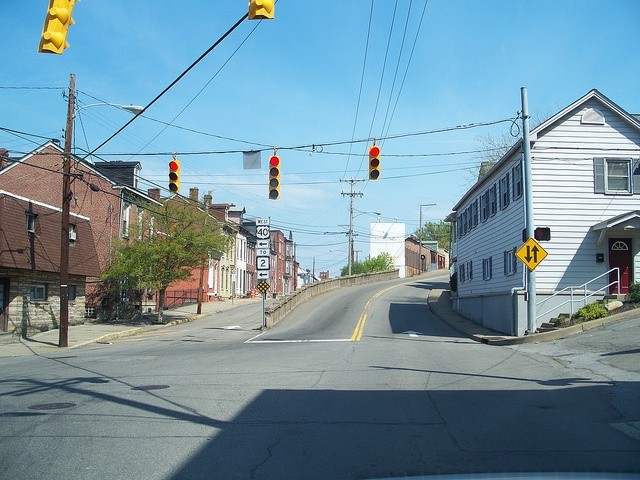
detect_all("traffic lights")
[366,144,381,181]
[167,160,181,195]
[267,153,281,202]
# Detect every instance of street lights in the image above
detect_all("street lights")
[58,71,145,347]
